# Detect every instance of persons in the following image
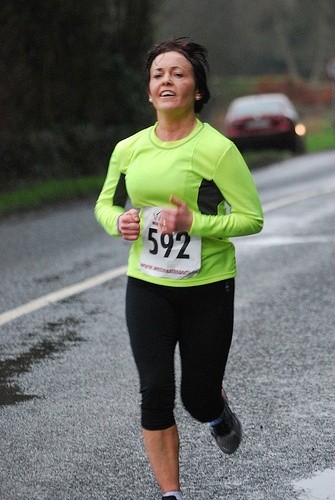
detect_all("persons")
[93,38,265,499]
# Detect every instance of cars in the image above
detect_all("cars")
[222,92,307,160]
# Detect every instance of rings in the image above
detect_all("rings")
[163,219,166,226]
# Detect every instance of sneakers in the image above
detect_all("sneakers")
[210,387,242,454]
